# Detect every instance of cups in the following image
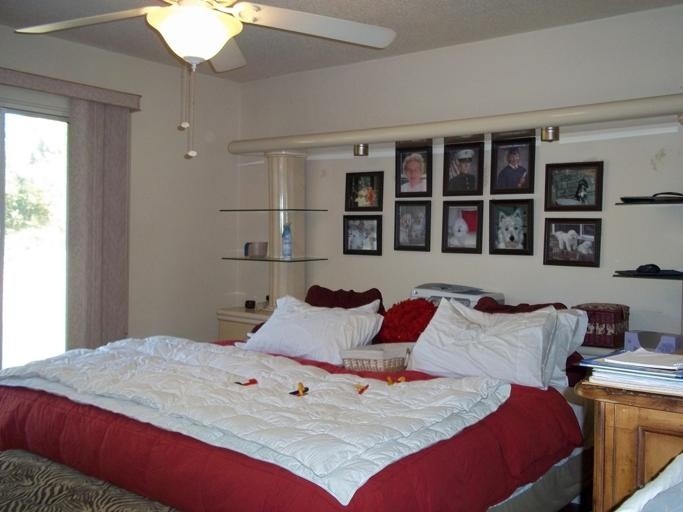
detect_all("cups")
[245,300,255,308]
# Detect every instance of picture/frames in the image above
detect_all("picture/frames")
[340,135,606,270]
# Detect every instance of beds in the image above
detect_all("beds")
[0,331,597,511]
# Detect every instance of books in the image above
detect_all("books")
[579,350,683,400]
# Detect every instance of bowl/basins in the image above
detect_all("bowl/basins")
[248,242,267,258]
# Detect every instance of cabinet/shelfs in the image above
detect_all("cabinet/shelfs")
[608,198,682,283]
[214,205,329,267]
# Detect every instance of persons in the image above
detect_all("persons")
[497,148,529,191]
[450,148,480,192]
[400,154,428,194]
[349,221,370,249]
[354,185,378,207]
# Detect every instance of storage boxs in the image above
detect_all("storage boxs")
[571,299,633,352]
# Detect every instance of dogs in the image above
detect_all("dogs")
[451,217,473,249]
[576,241,593,255]
[351,229,363,249]
[400,213,415,245]
[495,208,524,250]
[554,229,578,252]
[574,179,589,204]
[367,232,377,250]
[410,211,426,242]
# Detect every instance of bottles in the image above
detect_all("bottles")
[281,223,292,258]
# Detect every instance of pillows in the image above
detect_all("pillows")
[240,294,383,366]
[406,295,555,391]
[547,308,588,394]
[304,285,385,344]
[474,296,568,317]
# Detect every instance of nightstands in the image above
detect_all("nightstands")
[214,297,275,344]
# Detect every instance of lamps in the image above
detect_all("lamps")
[135,1,247,163]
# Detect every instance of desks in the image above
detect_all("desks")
[568,376,683,512]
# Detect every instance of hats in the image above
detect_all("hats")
[455,149,474,163]
[497,144,525,155]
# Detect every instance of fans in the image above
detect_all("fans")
[11,1,399,76]
